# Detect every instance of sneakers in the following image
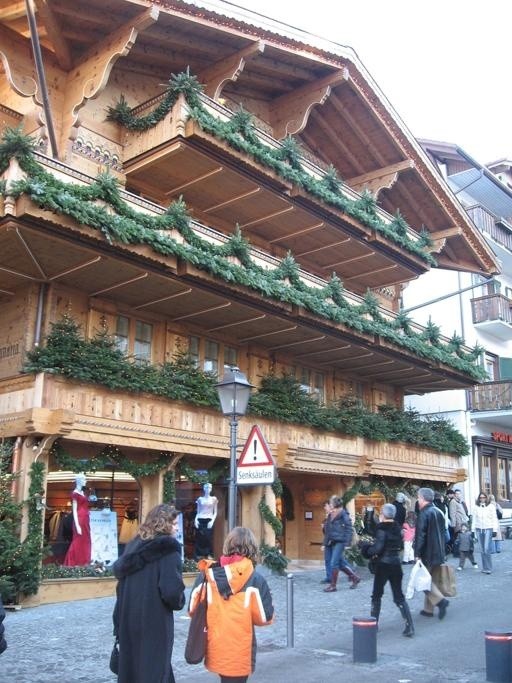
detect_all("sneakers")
[457,567,463,570]
[473,565,478,568]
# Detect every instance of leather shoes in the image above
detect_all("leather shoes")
[420,610,433,617]
[436,598,449,619]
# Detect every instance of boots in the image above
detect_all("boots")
[342,566,361,589]
[397,601,415,636]
[323,569,338,592]
[371,599,381,632]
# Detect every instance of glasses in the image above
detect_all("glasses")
[479,497,486,499]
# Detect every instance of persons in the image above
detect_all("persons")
[187,527,274,683]
[194,483,219,561]
[62,474,91,566]
[113,503,185,683]
[321,487,502,638]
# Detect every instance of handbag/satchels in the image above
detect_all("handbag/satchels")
[495,503,502,519]
[430,565,456,597]
[110,639,120,675]
[185,574,207,664]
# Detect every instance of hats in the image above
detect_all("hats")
[446,490,455,495]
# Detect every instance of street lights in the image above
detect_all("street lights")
[212,365,256,529]
